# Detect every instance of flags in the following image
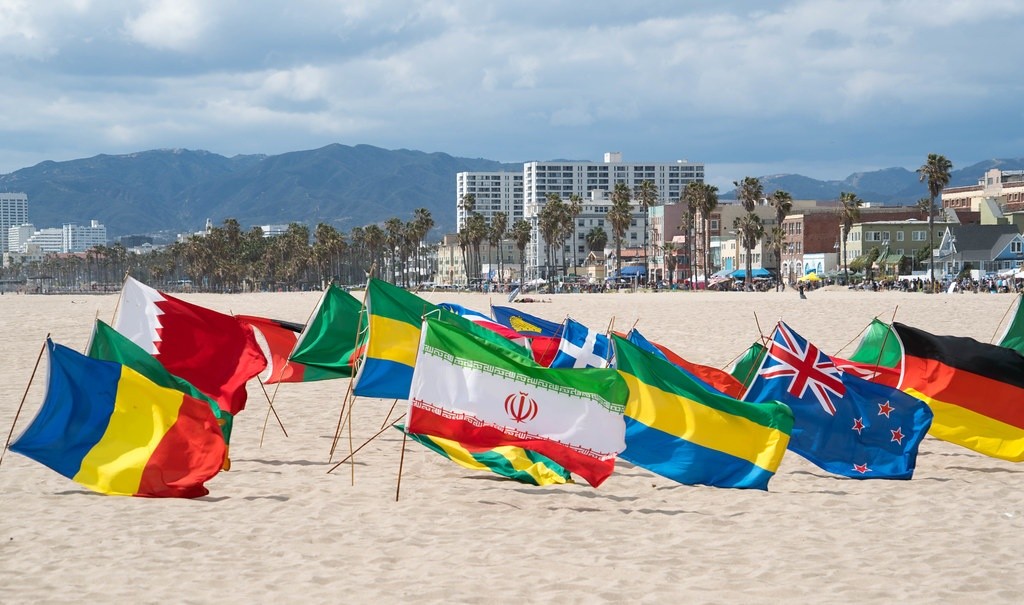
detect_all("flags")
[8,273,368,498]
[352,279,535,401]
[393,293,1024,489]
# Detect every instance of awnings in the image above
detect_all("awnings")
[614,266,648,277]
[712,268,770,277]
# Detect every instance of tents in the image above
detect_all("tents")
[798,272,821,283]
[1001,270,1024,278]
[528,278,549,285]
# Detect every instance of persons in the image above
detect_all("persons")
[935,274,1024,292]
[481,281,488,294]
[849,276,931,291]
[732,278,771,292]
